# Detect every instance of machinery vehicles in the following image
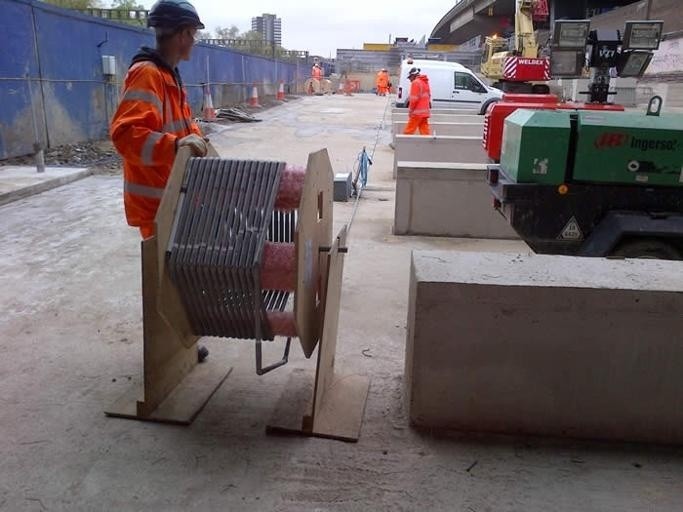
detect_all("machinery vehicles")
[482,19,683,261]
[479,0,551,94]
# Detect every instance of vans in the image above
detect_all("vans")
[395,58,505,116]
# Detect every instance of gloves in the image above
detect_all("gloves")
[177,131,208,157]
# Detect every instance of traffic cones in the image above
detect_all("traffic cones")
[198,84,224,122]
[276,79,285,100]
[247,82,262,108]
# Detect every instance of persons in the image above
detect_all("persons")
[388,71,431,151]
[408,66,431,109]
[108,0,209,364]
[378,68,387,97]
[375,68,385,95]
[311,62,321,81]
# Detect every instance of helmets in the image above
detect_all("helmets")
[147,0,205,29]
[406,68,420,81]
